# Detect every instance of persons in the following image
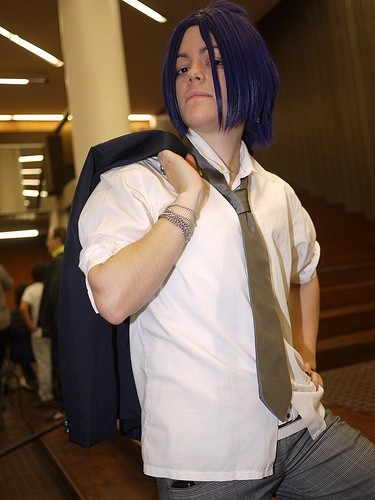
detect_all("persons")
[0,227,69,422]
[76,0,375,496]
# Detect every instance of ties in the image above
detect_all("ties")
[199,168,293,424]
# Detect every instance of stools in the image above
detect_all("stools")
[0,371,23,418]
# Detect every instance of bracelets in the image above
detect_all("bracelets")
[158,203,199,243]
